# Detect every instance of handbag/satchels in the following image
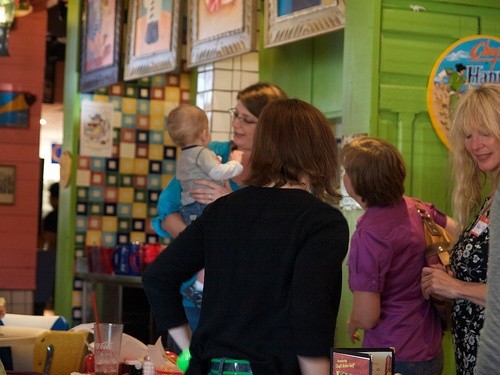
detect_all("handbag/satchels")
[413,198,453,320]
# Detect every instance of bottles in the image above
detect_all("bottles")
[142,355,155,375]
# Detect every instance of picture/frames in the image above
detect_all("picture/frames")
[79,0,345,92]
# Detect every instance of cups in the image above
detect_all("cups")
[111,244,141,276]
[93,322,125,375]
[130,242,161,275]
[86,245,114,275]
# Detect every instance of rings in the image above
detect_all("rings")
[209,194,211,199]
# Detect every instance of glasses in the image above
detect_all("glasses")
[229,107,257,125]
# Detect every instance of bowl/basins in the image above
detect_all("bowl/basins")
[0,326,50,375]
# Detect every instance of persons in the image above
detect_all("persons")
[168,103,244,310]
[143,98,349,375]
[421,83,500,375]
[343,137,466,375]
[38,183,59,250]
[152,82,289,334]
